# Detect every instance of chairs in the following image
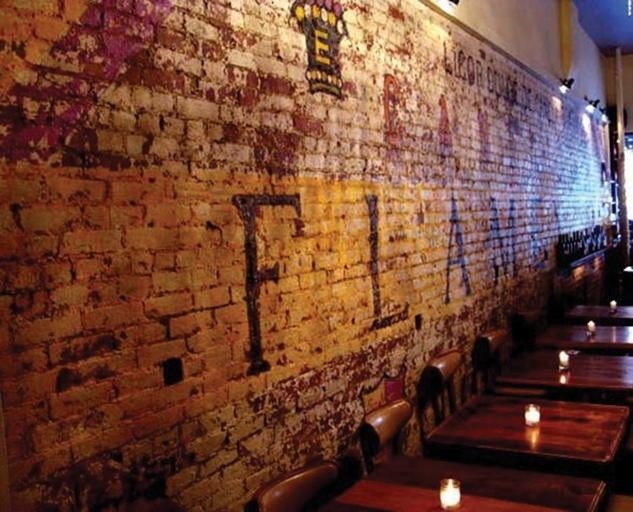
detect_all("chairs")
[414,347,470,458]
[359,396,413,476]
[469,328,515,395]
[508,305,554,359]
[250,461,338,512]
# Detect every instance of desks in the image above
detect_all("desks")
[538,323,633,351]
[334,456,610,511]
[423,390,631,481]
[494,349,633,394]
[567,304,633,324]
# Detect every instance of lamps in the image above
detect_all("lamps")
[558,74,612,124]
